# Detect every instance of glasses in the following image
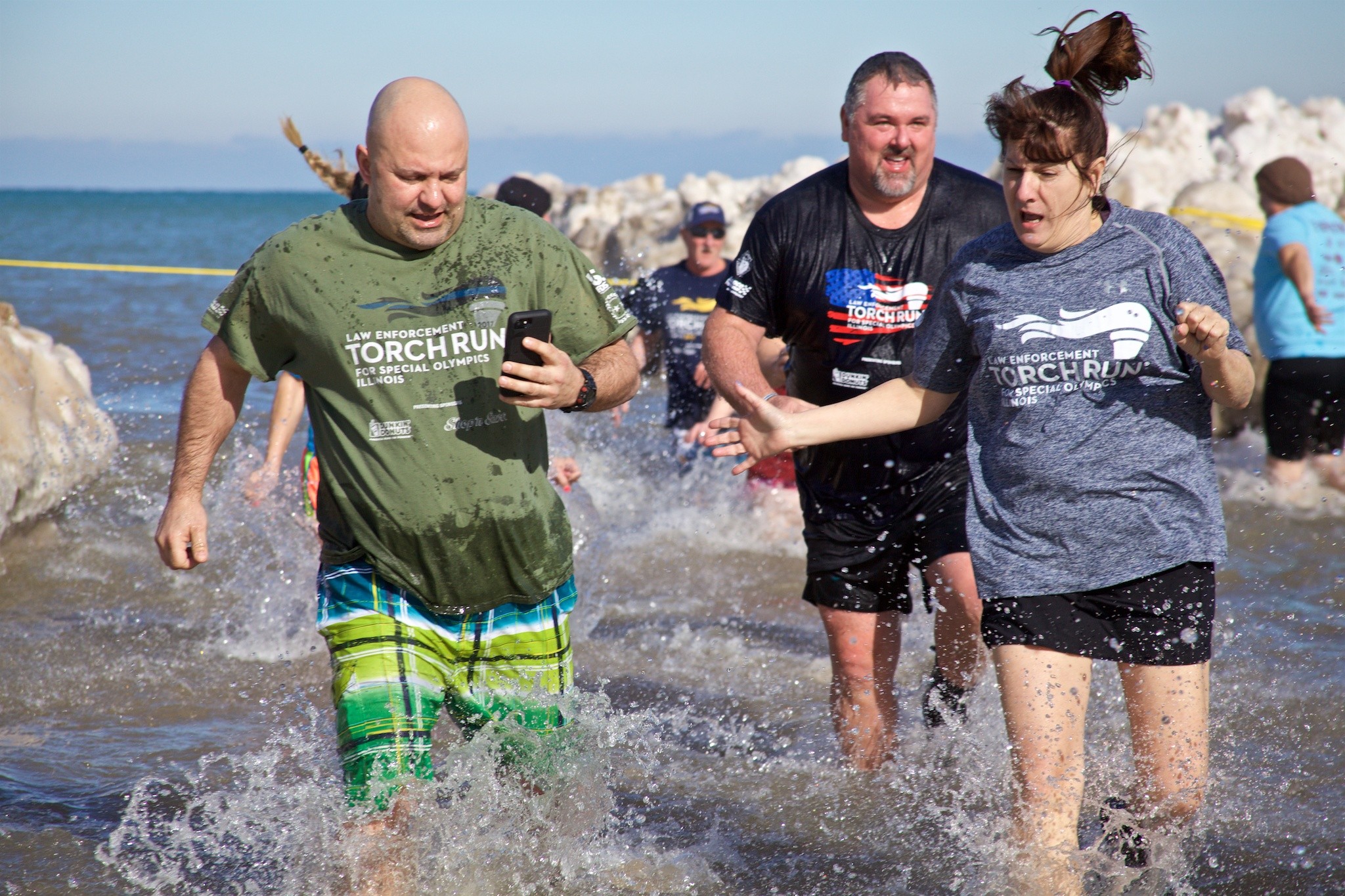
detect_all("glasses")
[685,224,727,240]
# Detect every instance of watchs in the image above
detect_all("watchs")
[559,367,596,414]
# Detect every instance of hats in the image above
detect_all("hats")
[683,201,727,230]
[497,178,552,217]
[1254,158,1315,204]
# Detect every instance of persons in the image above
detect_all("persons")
[628,201,734,477]
[1254,156,1345,493]
[702,9,1254,896]
[493,176,555,226]
[245,169,581,512]
[686,336,799,490]
[154,79,644,896]
[703,51,1012,767]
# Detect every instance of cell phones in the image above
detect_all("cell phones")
[498,309,552,399]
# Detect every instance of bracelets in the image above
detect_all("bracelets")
[763,393,776,400]
